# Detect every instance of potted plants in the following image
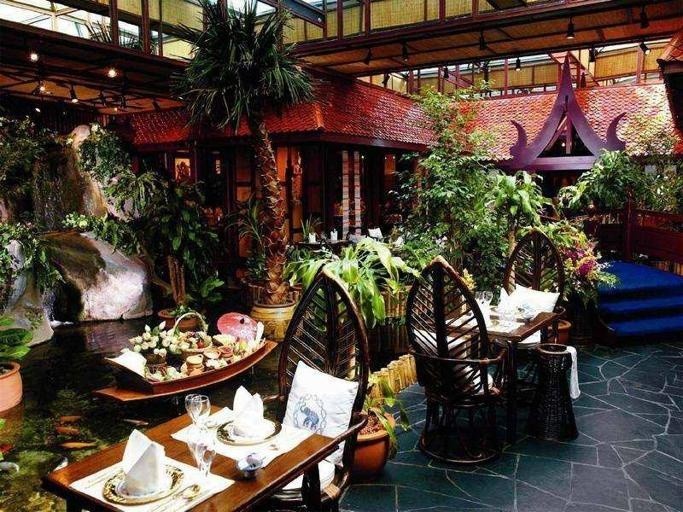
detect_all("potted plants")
[277,243,426,484]
[158,305,199,334]
[0,316,34,412]
[513,220,599,344]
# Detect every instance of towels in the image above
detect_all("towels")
[563,346,582,399]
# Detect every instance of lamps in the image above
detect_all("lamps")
[361,7,651,86]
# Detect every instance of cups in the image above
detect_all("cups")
[474,291,493,306]
[236,456,263,477]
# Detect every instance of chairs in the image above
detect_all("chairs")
[265,264,367,510]
[404,254,512,466]
[500,225,565,397]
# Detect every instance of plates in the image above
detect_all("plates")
[215,416,283,446]
[103,462,185,506]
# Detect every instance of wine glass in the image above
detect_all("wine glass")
[185,393,217,493]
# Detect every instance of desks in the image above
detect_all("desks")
[94,335,280,416]
[444,305,559,421]
[40,403,340,510]
